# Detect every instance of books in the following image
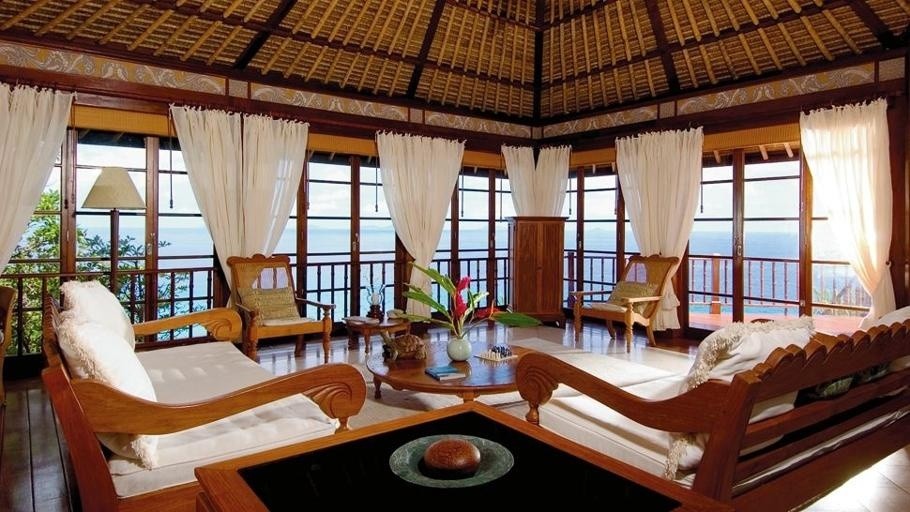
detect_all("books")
[340,315,381,326]
[424,364,467,382]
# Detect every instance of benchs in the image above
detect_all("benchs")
[516,319,909,511]
[42,293,367,510]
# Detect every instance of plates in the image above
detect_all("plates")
[386,433,515,490]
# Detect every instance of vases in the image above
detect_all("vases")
[447,338,471,361]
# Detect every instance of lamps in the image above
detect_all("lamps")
[81,166,147,294]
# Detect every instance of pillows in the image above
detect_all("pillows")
[55,280,160,472]
[662,314,813,477]
[870,303,909,398]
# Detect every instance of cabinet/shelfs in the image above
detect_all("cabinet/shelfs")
[504,216,569,328]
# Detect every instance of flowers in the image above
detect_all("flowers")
[393,261,544,338]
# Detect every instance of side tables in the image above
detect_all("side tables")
[194,400,737,512]
[342,316,412,354]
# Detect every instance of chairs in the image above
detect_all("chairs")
[570,254,680,353]
[0,286,16,406]
[226,253,336,364]
